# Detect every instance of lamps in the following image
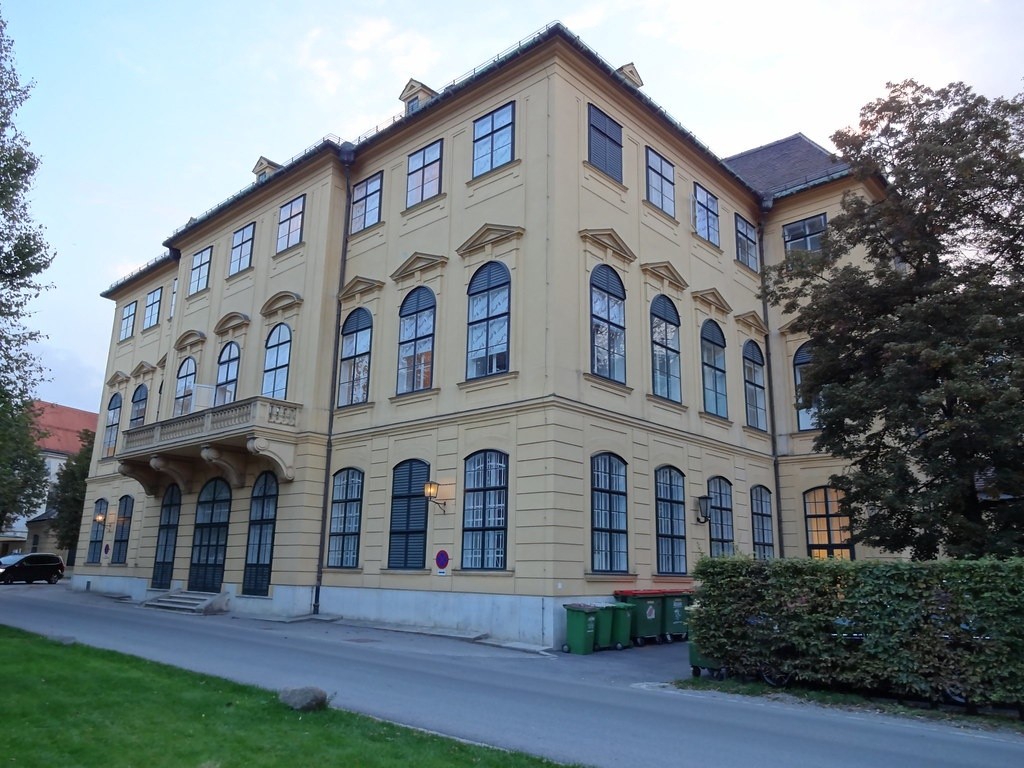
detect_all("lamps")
[424,481,447,515]
[697,495,713,524]
[93,512,112,531]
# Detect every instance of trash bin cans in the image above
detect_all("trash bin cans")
[614,588,664,647]
[562,603,598,655]
[588,602,616,651]
[660,588,700,643]
[610,602,637,650]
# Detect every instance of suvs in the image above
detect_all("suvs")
[0,553,65,585]
